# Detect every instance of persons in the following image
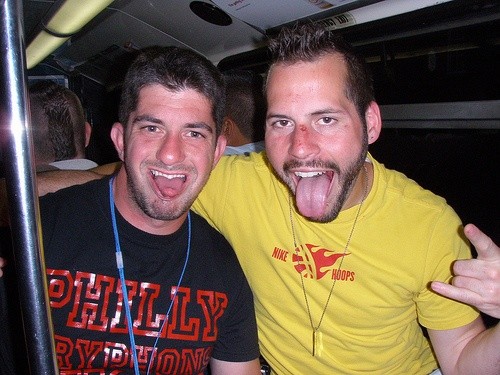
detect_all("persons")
[0,43,262,375]
[27,64,266,168]
[0,161,16,375]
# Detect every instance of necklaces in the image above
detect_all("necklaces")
[0,19,500,375]
[288,187,367,357]
